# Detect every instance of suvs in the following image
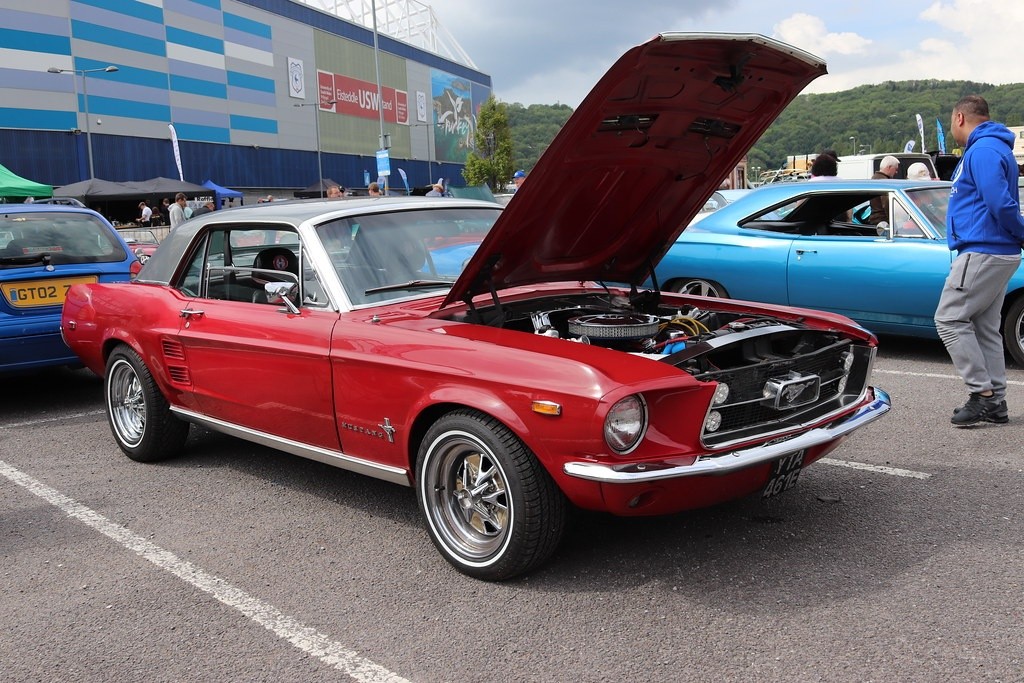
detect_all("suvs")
[935,153,962,181]
[0,197,143,373]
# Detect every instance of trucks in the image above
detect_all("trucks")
[1005,126,1024,176]
[786,154,820,170]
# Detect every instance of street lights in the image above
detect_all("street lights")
[415,122,444,184]
[752,166,761,187]
[292,101,338,197]
[48,65,120,180]
[858,145,871,155]
[849,136,856,156]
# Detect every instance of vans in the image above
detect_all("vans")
[835,153,941,181]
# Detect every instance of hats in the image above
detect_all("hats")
[139,202,145,207]
[511,171,524,179]
[822,150,841,162]
[433,184,443,190]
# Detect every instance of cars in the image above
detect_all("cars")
[60,25,892,584]
[594,179,1024,368]
[117,230,160,265]
[783,163,787,168]
[699,189,781,221]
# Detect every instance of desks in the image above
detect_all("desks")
[114,226,140,230]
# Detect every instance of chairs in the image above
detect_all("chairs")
[7,238,48,249]
[378,225,435,302]
[252,247,309,308]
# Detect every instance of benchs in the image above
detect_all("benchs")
[184,265,377,303]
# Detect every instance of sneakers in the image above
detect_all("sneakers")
[951,389,1004,425]
[950,400,1009,425]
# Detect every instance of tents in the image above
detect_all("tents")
[0,162,54,204]
[53,176,218,221]
[202,180,243,210]
[294,178,353,198]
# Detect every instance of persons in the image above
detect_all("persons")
[368,182,385,196]
[96,206,104,215]
[896,161,951,232]
[869,155,900,226]
[513,171,525,190]
[807,149,840,182]
[835,208,853,222]
[327,185,349,198]
[425,183,444,198]
[136,192,214,233]
[932,94,1024,428]
[257,195,274,203]
